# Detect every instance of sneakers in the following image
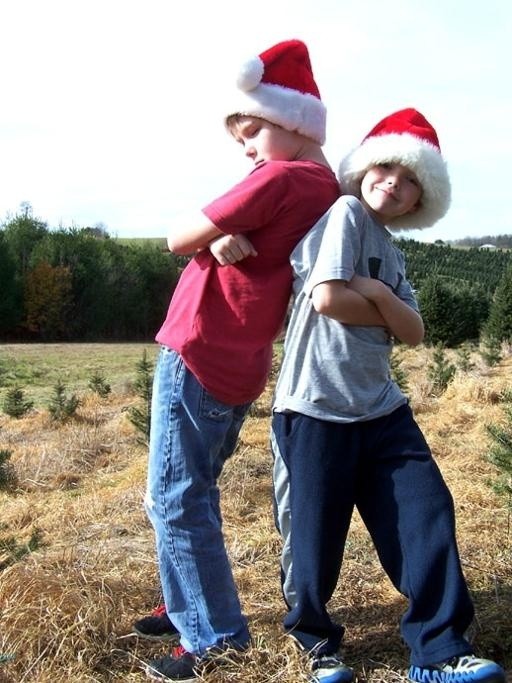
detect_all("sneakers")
[299,653,351,682]
[147,647,216,680]
[134,603,179,640]
[409,652,505,681]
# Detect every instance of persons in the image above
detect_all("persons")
[127,35,344,682]
[257,104,512,681]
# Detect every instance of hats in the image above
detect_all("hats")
[216,38,330,148]
[341,104,448,228]
